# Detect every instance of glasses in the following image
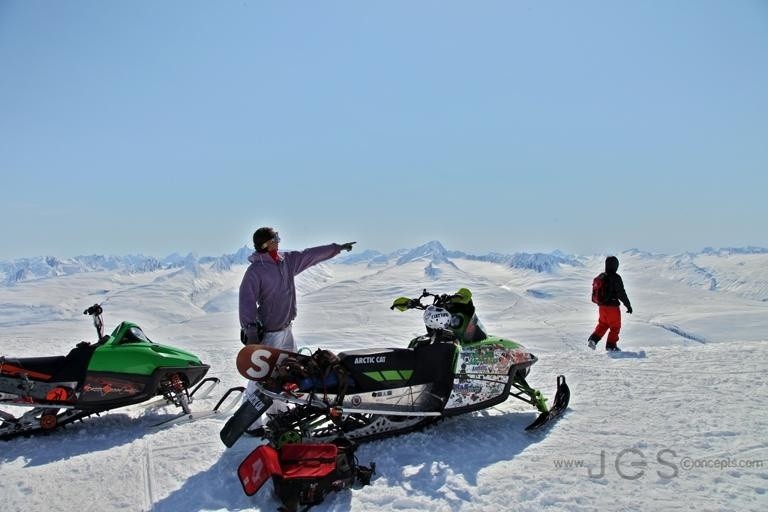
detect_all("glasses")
[262,232,281,248]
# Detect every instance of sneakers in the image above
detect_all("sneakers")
[244,427,266,437]
[606,347,622,352]
[587,339,596,350]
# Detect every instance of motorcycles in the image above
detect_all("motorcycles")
[219,286,568,455]
[2,302,245,445]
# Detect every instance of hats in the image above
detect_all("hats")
[253,226,276,253]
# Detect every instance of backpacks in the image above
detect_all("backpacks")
[592,272,612,305]
[238,436,356,508]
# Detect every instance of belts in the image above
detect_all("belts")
[265,320,291,333]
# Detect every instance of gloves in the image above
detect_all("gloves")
[626,307,632,313]
[341,242,357,251]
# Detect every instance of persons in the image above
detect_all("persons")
[584,256,632,352]
[236,226,358,437]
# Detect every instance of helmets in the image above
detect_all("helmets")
[240,320,265,344]
[423,304,452,331]
